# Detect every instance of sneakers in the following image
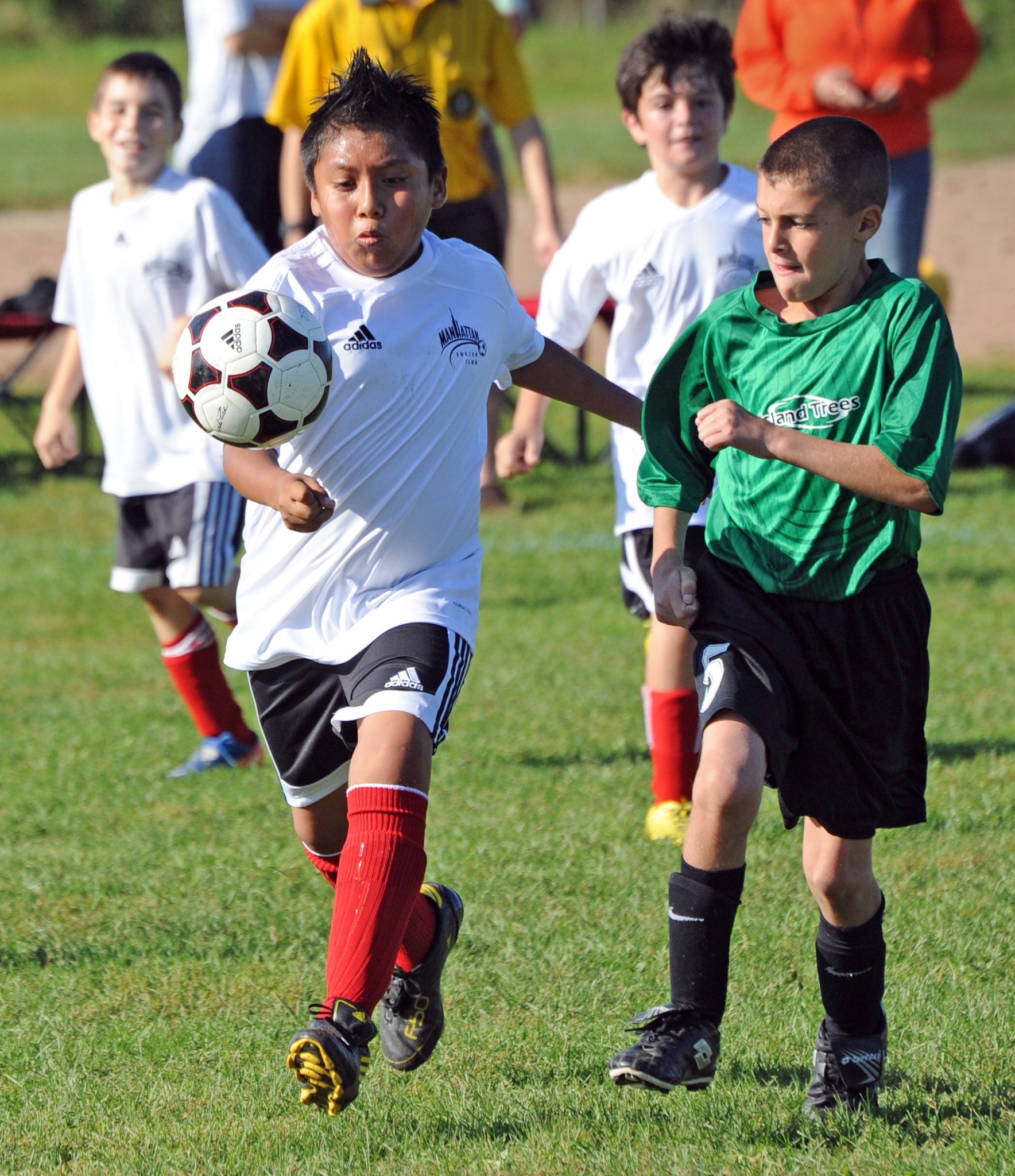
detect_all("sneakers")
[160,729,263,774]
[378,881,465,1068]
[801,1011,889,1125]
[608,1002,720,1091]
[645,798,693,848]
[286,996,378,1119]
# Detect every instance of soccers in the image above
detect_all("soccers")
[168,288,336,450]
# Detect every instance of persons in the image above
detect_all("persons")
[601,116,966,1116]
[261,0,564,298]
[729,0,984,283]
[175,0,309,262]
[34,53,275,781]
[493,14,772,848]
[220,50,722,1118]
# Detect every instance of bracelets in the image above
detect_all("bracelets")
[277,219,309,238]
